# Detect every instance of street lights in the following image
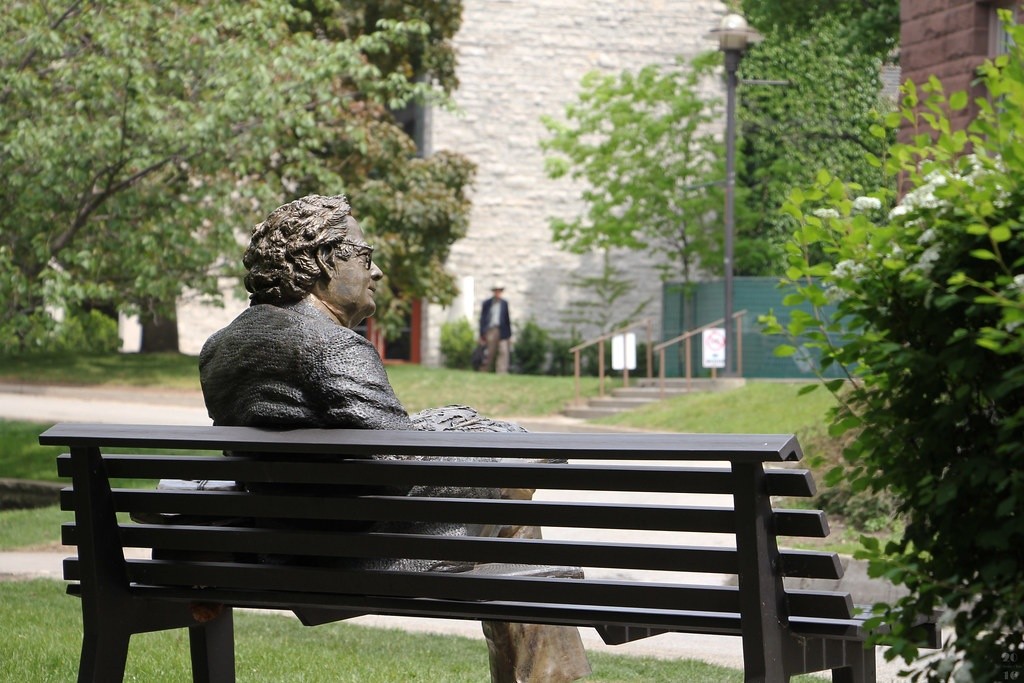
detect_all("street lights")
[701,13,766,376]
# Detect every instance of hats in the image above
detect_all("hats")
[492,281,505,291]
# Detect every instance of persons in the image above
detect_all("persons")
[479,284,512,374]
[199,195,595,683]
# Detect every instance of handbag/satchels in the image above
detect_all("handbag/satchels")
[470,343,488,370]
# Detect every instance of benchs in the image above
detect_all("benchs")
[39,420,943,683]
